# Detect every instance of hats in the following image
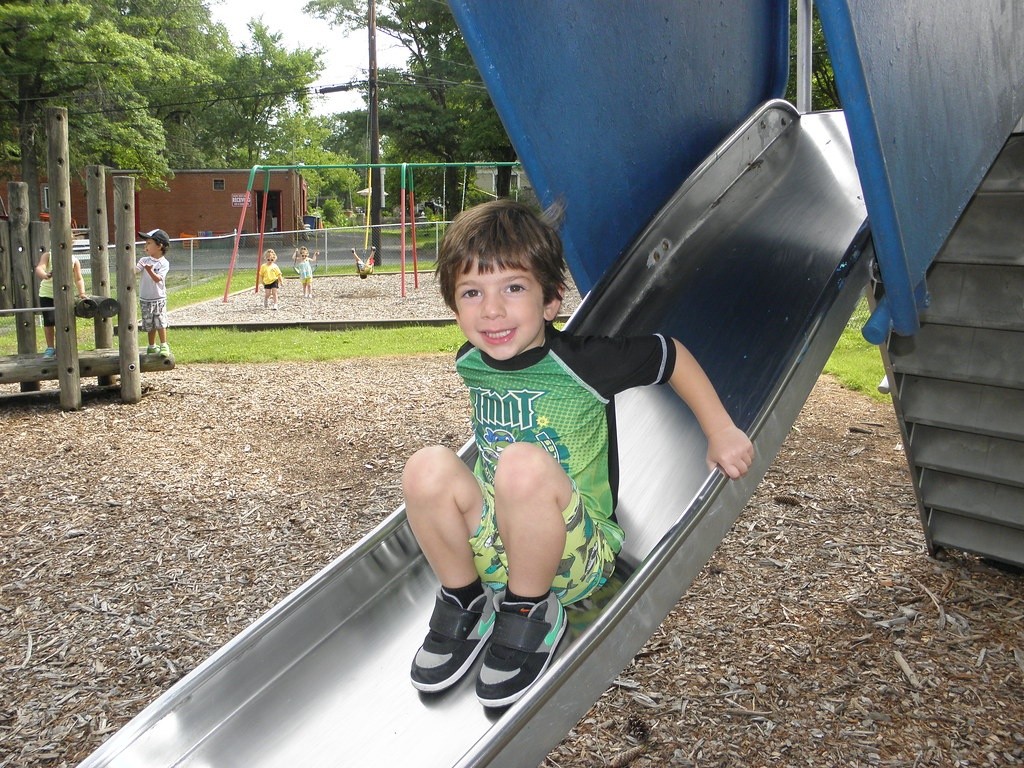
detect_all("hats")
[138,228,170,247]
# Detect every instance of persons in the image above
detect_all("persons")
[292,246,319,298]
[135,229,170,356]
[36,233,86,362]
[260,249,284,310]
[402,200,754,708]
[352,247,376,279]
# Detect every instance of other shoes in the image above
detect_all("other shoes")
[371,247,376,251]
[264,300,270,308]
[309,294,313,298]
[351,249,354,254]
[304,294,307,297]
[273,304,278,309]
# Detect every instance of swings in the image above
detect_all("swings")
[292,170,319,274]
[346,168,371,272]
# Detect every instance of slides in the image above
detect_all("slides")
[72,99,871,768]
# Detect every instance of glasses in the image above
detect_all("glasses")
[301,255,307,258]
[266,258,274,261]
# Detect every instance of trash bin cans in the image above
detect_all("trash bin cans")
[303,215,320,232]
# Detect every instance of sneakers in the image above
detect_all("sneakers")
[43,347,56,360]
[410,583,497,691]
[147,345,160,353]
[476,590,568,708]
[159,345,169,357]
